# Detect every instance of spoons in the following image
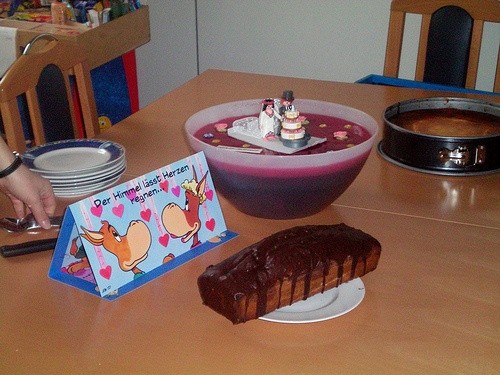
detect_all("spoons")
[0,212,64,233]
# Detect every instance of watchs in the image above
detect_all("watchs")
[0,150,22,179]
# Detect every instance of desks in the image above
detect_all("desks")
[0,4,152,142]
[0,67,500,375]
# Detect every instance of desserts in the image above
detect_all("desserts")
[197,221,384,323]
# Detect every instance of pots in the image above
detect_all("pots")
[376,96,500,177]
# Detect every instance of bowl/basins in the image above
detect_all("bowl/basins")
[185,98,379,220]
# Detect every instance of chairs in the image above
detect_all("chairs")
[0,33,103,157]
[381,0,500,95]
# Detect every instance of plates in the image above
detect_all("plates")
[21,139,128,198]
[258,277,366,324]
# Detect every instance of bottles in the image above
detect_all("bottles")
[51,1,67,26]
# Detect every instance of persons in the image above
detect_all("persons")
[0,136,57,229]
[259,91,294,141]
